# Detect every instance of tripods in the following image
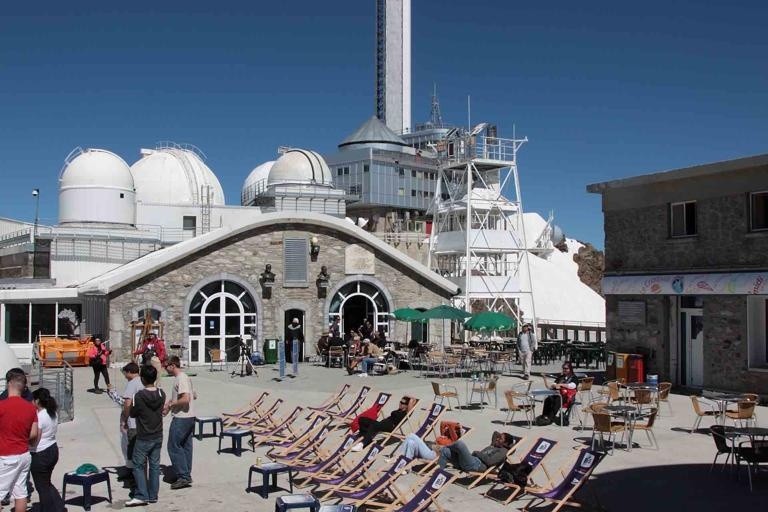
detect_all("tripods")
[231,346,257,377]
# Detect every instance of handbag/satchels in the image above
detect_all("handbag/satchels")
[90,356,102,367]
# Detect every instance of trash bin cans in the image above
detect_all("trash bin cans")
[607,350,645,384]
[265,339,279,364]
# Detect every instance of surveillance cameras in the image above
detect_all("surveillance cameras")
[32,190,38,197]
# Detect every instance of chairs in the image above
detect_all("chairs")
[38,333,91,367]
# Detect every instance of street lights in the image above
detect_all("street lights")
[31,188,39,233]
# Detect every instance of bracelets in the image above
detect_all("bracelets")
[168,400,172,409]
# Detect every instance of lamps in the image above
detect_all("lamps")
[310,237,321,256]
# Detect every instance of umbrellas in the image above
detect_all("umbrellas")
[388,307,427,352]
[419,304,472,355]
[463,310,517,362]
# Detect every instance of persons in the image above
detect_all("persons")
[162,354,197,488]
[120,361,147,485]
[516,322,539,380]
[350,396,416,454]
[285,315,387,377]
[1,367,68,512]
[535,361,579,426]
[430,430,514,471]
[383,420,462,475]
[132,331,167,369]
[124,364,166,508]
[88,335,117,394]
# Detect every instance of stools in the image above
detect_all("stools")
[63,468,113,511]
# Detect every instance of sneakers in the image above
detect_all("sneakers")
[125,499,148,507]
[171,478,189,489]
